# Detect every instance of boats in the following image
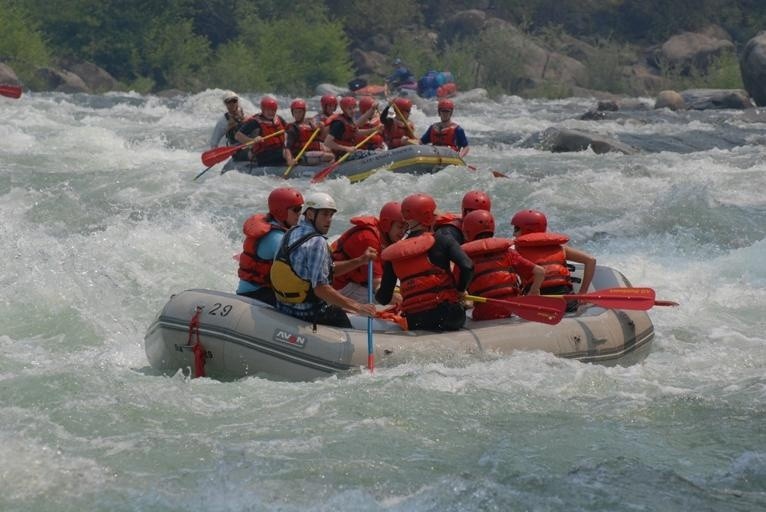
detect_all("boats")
[219,145,466,179]
[142,248,656,384]
[321,79,491,113]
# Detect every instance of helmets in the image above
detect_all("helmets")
[267,188,304,223]
[401,194,436,226]
[393,98,412,110]
[261,96,278,110]
[321,95,376,114]
[511,210,547,237]
[223,92,239,102]
[462,192,490,217]
[392,58,402,65]
[380,202,405,233]
[302,193,337,216]
[438,100,453,116]
[291,99,305,112]
[463,210,495,242]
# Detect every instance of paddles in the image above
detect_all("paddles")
[309,130,380,185]
[202,129,285,166]
[392,288,567,326]
[538,287,656,311]
[404,137,509,177]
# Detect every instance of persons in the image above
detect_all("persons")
[270,194,379,327]
[329,201,409,305]
[452,209,545,322]
[237,187,305,307]
[376,194,475,331]
[511,209,597,312]
[434,192,491,245]
[209,58,469,166]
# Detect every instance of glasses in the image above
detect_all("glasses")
[514,226,520,233]
[288,206,302,213]
[227,99,238,103]
[404,108,410,112]
